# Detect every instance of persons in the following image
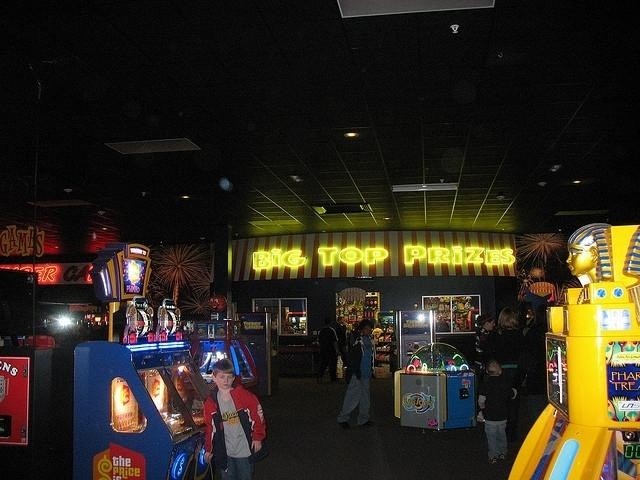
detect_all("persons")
[563,224,611,303]
[336,318,376,430]
[474,306,544,464]
[316,318,360,385]
[202,357,266,479]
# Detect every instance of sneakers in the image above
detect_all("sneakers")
[489,456,498,464]
[498,453,505,464]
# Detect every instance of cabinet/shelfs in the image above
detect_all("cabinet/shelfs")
[336,291,381,330]
[372,333,397,379]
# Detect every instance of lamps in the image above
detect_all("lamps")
[391,183,460,193]
